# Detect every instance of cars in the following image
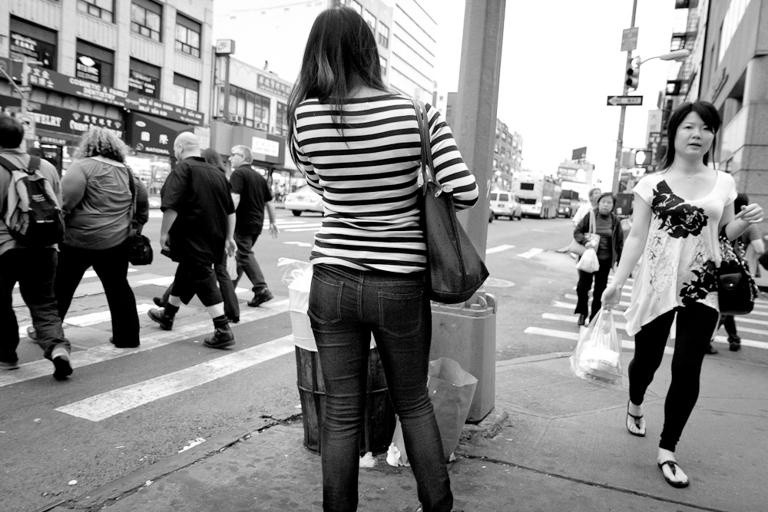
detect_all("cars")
[488,207,495,224]
[280,183,327,218]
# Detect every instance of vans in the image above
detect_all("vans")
[488,190,523,221]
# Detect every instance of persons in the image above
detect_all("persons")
[573,188,602,229]
[26,127,149,350]
[284,6,481,512]
[0,116,75,380]
[706,192,766,354]
[147,132,279,349]
[573,192,624,326]
[601,99,764,489]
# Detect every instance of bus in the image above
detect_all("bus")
[513,172,562,220]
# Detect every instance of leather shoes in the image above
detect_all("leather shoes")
[148,297,173,330]
[248,289,273,307]
[204,325,235,348]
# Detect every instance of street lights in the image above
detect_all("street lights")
[610,49,692,196]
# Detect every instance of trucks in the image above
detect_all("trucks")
[559,189,581,219]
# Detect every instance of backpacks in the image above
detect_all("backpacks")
[0,155,64,246]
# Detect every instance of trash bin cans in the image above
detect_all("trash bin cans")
[288,284,396,457]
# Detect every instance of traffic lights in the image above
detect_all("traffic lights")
[605,94,643,107]
[622,146,652,169]
[625,55,641,89]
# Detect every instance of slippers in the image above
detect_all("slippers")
[626,400,646,437]
[658,461,689,488]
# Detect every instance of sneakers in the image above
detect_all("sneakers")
[51,347,73,379]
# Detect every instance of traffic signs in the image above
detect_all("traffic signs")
[11,84,33,94]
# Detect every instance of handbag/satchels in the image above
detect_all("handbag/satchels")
[570,232,600,255]
[418,182,489,304]
[129,235,152,265]
[720,264,758,315]
